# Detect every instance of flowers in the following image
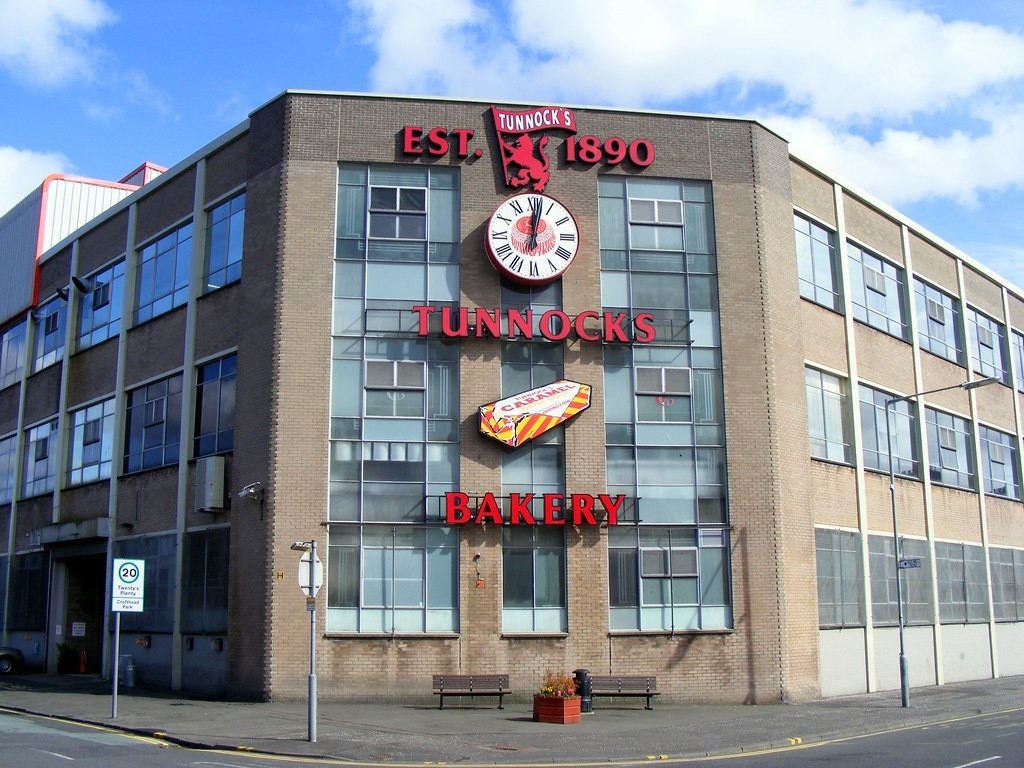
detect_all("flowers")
[539,668,580,698]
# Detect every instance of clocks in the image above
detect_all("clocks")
[484,192,580,285]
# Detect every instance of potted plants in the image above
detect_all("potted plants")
[55,641,82,674]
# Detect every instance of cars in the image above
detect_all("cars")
[0,645,25,676]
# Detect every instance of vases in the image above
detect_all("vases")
[533,693,582,724]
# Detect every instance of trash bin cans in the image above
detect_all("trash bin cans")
[573,669,592,712]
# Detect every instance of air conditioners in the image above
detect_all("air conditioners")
[195,456,225,510]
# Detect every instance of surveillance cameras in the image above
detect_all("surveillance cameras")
[238,488,255,497]
[475,552,481,558]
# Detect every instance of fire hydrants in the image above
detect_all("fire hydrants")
[573,667,594,713]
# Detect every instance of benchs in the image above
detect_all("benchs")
[574,675,661,710]
[433,674,512,710]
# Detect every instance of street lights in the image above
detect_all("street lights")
[886,375,1003,707]
[291,539,318,743]
